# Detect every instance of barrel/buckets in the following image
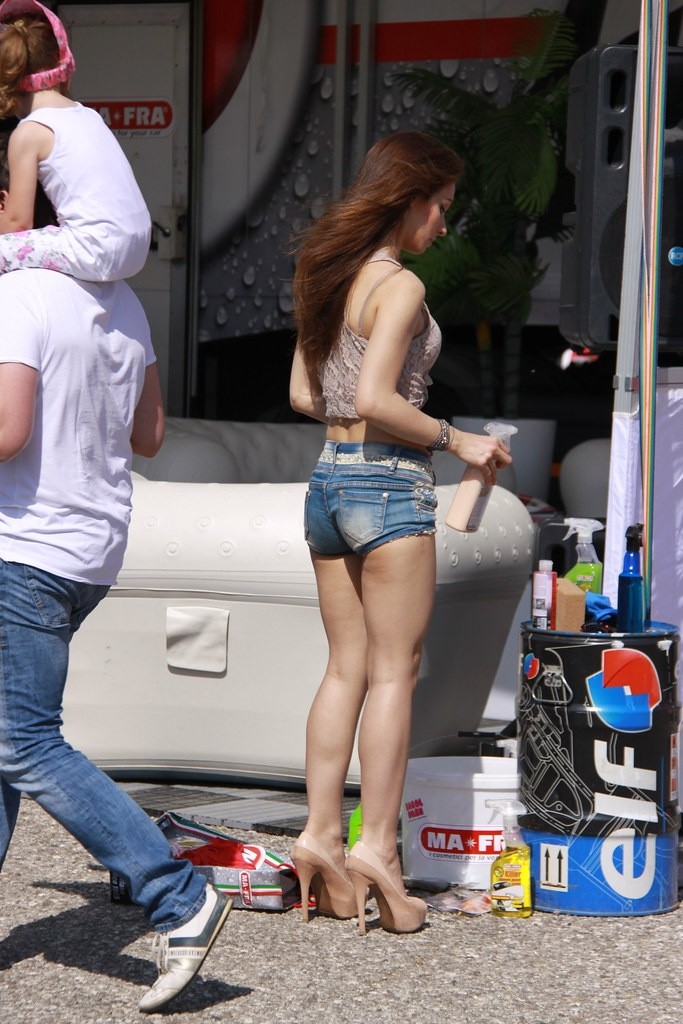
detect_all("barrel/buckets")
[402,757,520,889]
[519,620,681,916]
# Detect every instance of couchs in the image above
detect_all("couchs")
[60,412,556,803]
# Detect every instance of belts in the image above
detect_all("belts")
[320,450,433,476]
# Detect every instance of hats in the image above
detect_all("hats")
[0,0,76,92]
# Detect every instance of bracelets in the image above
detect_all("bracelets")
[429,419,455,452]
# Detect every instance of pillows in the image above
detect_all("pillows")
[437,416,556,504]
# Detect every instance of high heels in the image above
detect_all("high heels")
[344,840,427,937]
[290,833,371,922]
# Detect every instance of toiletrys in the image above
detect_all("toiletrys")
[616,522,645,634]
[531,558,558,630]
[561,516,605,594]
[446,421,519,534]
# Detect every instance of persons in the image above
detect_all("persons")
[292,130,513,934]
[0,0,151,280]
[0,131,233,1008]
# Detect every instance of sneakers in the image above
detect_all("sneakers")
[137,883,234,1010]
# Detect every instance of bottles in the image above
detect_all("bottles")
[532,560,557,629]
[444,422,518,532]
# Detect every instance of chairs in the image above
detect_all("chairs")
[535,516,606,578]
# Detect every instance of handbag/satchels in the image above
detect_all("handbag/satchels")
[107,811,316,912]
